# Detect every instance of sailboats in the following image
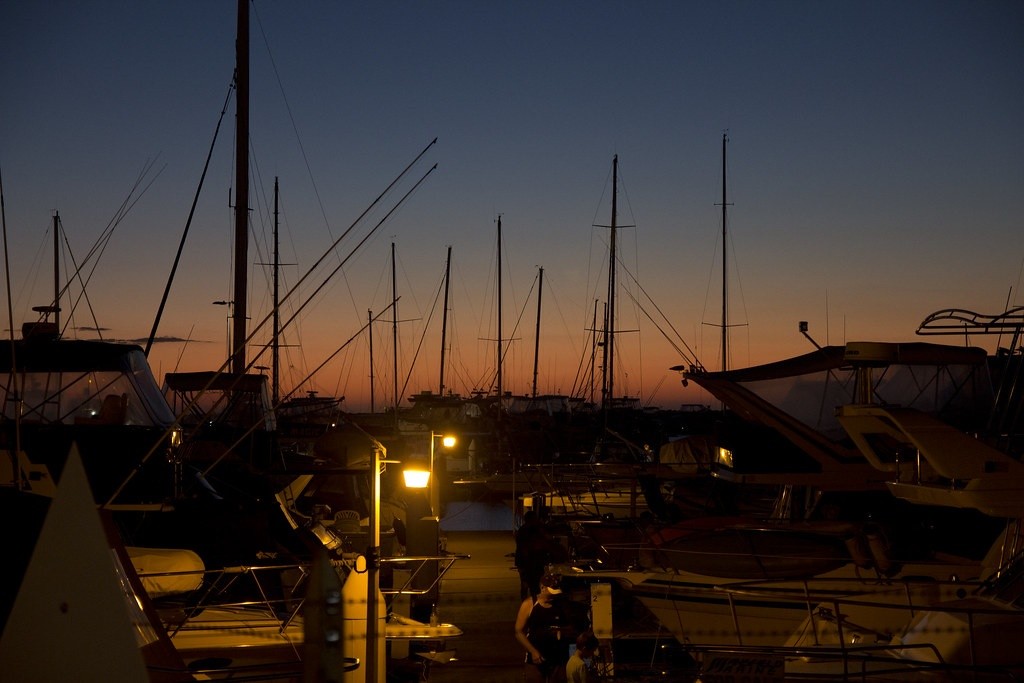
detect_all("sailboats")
[0,0,1024,683]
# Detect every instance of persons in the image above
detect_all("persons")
[566,631,600,683]
[515,574,568,683]
[515,510,546,602]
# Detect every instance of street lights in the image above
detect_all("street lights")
[429,430,455,517]
[370,446,432,683]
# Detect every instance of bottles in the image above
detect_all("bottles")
[392,511,406,547]
[429,603,437,628]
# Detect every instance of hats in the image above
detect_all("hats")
[540,574,562,594]
